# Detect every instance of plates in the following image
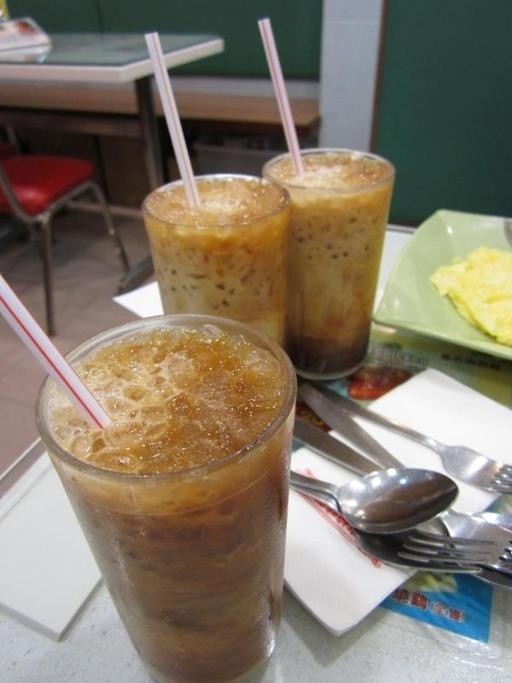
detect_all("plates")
[370,208,512,361]
[281,366,512,638]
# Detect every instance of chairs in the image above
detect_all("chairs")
[1,150,136,341]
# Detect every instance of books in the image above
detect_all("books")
[0,15,50,50]
[0,44,52,63]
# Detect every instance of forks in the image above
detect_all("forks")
[287,471,499,582]
[295,386,512,592]
[299,376,511,495]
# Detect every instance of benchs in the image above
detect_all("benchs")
[0,81,319,141]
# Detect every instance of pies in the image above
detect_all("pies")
[429,243,512,346]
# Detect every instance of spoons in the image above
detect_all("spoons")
[285,468,460,535]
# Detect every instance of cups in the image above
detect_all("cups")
[140,172,292,351]
[33,313,299,683]
[260,147,395,384]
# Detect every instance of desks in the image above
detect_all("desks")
[1,30,229,212]
